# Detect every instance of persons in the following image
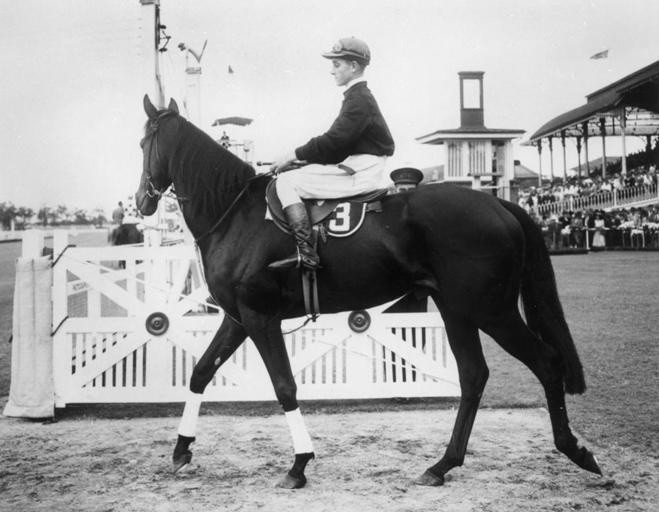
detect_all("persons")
[112,193,146,232]
[384,167,427,403]
[517,163,659,248]
[267,36,397,272]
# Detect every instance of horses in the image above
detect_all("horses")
[133,92,604,489]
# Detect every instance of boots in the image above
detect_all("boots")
[268,203,320,269]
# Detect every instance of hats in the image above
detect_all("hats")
[390,167,423,185]
[321,36,371,61]
